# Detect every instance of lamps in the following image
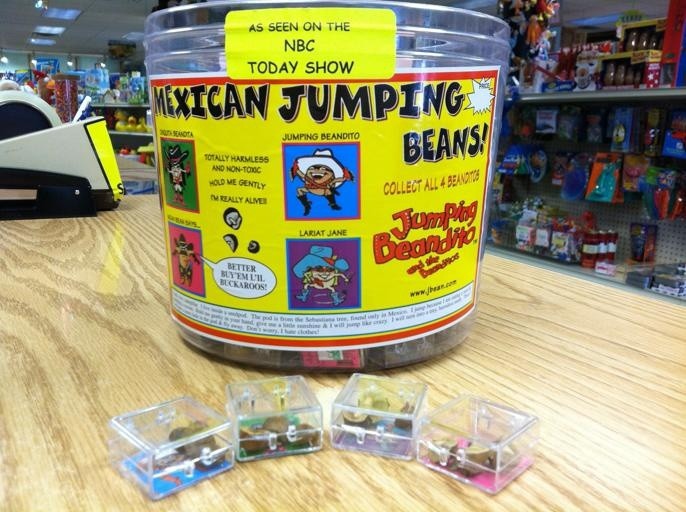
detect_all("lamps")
[0,47,111,69]
[36,0,48,11]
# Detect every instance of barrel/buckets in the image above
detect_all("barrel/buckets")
[142,2,509,371]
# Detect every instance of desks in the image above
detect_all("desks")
[0,155,686,512]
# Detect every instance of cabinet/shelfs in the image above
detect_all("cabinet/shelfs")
[86,100,156,165]
[487,79,686,309]
